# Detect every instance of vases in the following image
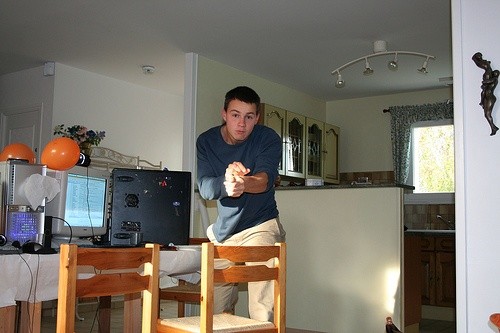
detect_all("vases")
[75,148,91,166]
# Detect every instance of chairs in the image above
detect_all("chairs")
[56,243,287,333]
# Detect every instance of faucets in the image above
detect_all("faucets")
[436,214,454,230]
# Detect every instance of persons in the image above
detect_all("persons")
[195,85,285,325]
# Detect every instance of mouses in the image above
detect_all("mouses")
[24,241,43,252]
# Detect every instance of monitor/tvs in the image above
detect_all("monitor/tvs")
[45,163,109,247]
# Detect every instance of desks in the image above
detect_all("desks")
[0,245,202,333]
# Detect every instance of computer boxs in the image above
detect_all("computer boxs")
[106,167,191,247]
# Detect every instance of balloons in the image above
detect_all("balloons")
[0,143,34,164]
[40,137,80,171]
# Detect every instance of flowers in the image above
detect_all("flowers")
[53,124,106,156]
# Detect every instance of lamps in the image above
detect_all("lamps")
[363,57,374,74]
[336,70,345,89]
[388,52,398,71]
[417,57,430,74]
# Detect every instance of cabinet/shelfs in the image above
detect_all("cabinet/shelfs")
[306,117,341,184]
[421,234,456,307]
[258,103,306,179]
